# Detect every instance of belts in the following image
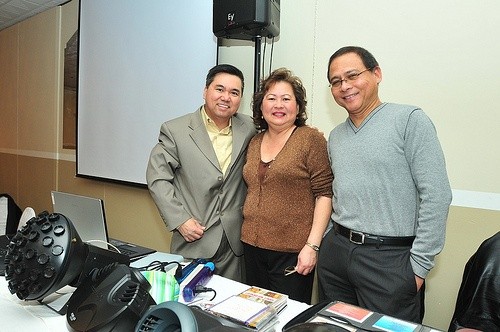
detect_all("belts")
[331,220,416,247]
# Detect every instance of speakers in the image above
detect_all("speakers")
[212,0,280,40]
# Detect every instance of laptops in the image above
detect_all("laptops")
[51,191,157,259]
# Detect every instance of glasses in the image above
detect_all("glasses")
[328,66,371,88]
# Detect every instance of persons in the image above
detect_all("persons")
[317,47,452,324]
[240,67,334,305]
[146,64,259,284]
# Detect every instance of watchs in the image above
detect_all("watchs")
[305,242,319,252]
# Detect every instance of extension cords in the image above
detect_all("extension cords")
[173,258,203,283]
[182,263,216,302]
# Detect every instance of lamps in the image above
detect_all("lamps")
[0,194,257,332]
